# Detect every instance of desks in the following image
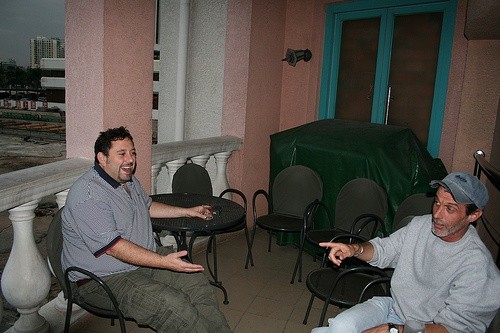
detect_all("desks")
[149,192,244,305]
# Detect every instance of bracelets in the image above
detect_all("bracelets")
[354,243,364,256]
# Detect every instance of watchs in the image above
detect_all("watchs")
[387,322,399,333]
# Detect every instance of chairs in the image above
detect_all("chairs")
[172,163,254,284]
[244,165,435,327]
[46,206,127,333]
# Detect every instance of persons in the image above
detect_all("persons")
[61,126,233,333]
[311,171,500,333]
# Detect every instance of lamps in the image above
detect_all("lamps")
[281,48,313,67]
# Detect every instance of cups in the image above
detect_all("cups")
[403,320,426,333]
[202,203,213,221]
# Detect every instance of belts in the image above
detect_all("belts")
[76,278,91,287]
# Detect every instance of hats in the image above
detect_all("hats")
[430,171,489,209]
